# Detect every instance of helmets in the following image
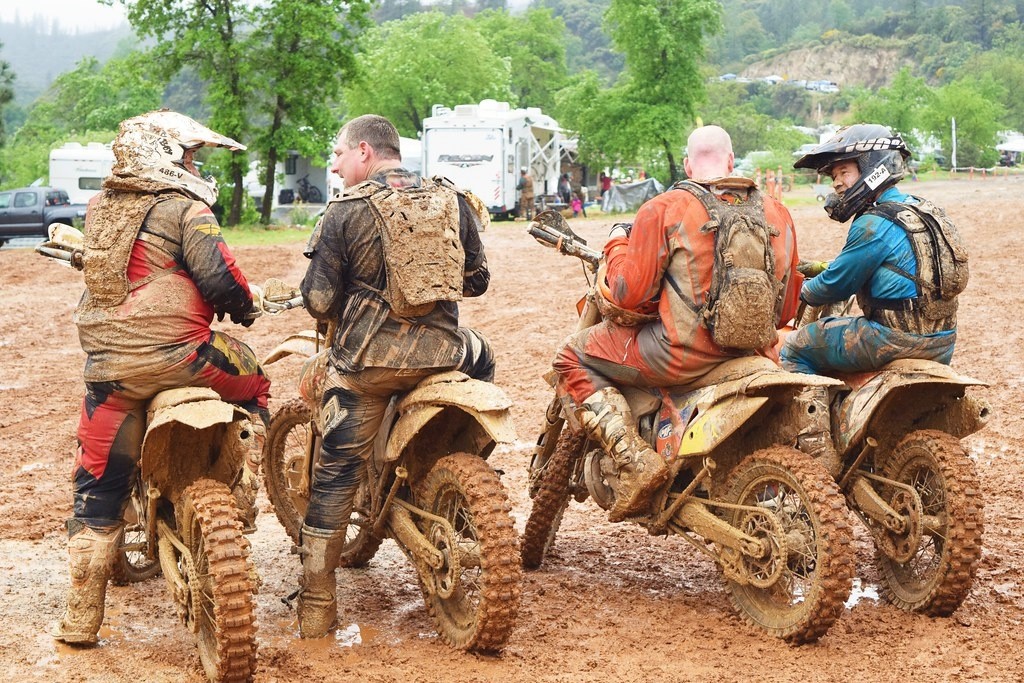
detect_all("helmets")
[793,123,912,223]
[521,167,528,174]
[594,259,661,327]
[111,108,247,208]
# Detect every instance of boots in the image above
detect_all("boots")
[233,414,267,527]
[798,385,843,478]
[574,386,669,524]
[298,523,347,640]
[51,516,125,646]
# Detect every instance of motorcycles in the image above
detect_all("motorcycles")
[775,257,993,618]
[765,177,791,192]
[520,211,856,644]
[262,278,524,655]
[34,223,286,683]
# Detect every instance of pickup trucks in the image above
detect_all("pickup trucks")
[0,186,89,247]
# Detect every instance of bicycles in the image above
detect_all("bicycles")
[296,174,322,202]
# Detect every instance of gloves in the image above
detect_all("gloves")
[797,257,828,277]
[216,310,255,328]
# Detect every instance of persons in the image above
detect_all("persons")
[551,125,805,522]
[627,169,633,183]
[779,122,958,373]
[297,115,496,637]
[52,107,271,643]
[554,173,588,218]
[599,172,611,199]
[516,167,536,221]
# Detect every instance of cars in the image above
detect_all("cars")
[905,146,946,167]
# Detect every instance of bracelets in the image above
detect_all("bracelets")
[609,225,628,238]
